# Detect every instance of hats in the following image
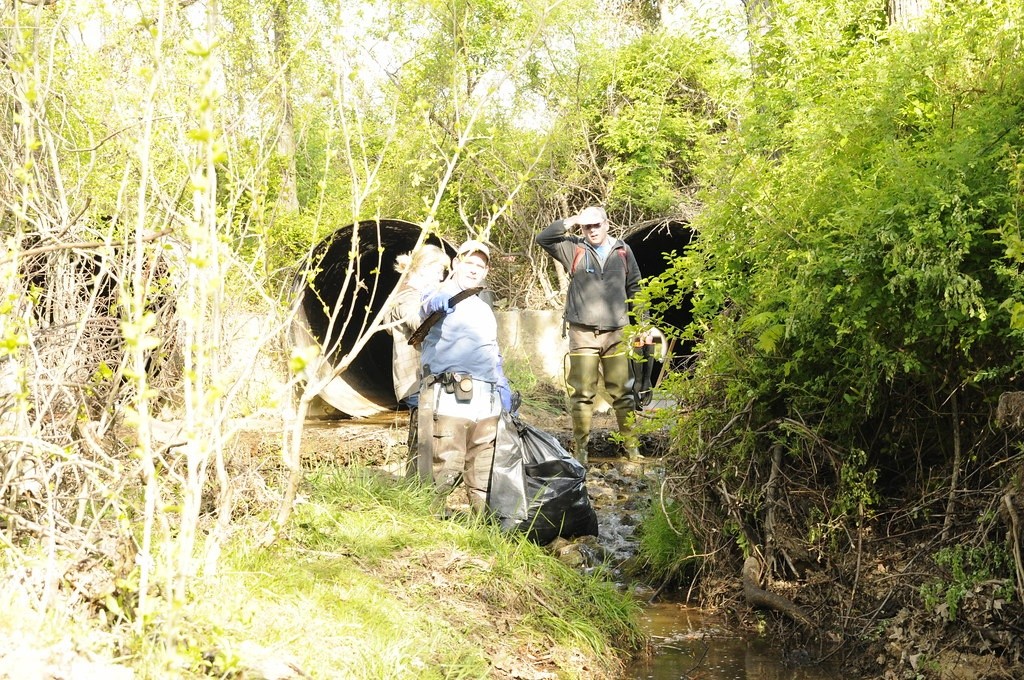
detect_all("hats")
[457,240,490,265]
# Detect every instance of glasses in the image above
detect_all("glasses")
[582,221,603,230]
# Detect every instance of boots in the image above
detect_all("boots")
[569,354,600,471]
[602,352,656,466]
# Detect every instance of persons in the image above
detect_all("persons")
[419,241,520,527]
[384,244,451,479]
[536,206,653,470]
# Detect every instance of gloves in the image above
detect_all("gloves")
[429,292,457,315]
[501,393,520,418]
[633,344,655,410]
